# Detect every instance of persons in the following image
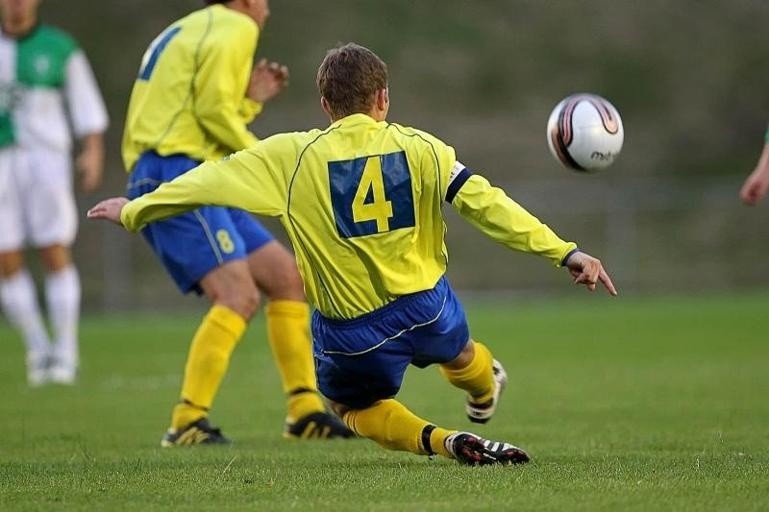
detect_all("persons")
[0,0,111,389]
[739,126,769,206]
[87,41,618,468]
[121,0,358,447]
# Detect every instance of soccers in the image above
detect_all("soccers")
[546,92,624,171]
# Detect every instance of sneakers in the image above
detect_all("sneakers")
[442,431,530,465]
[465,358,509,426]
[160,416,231,448]
[282,410,355,440]
